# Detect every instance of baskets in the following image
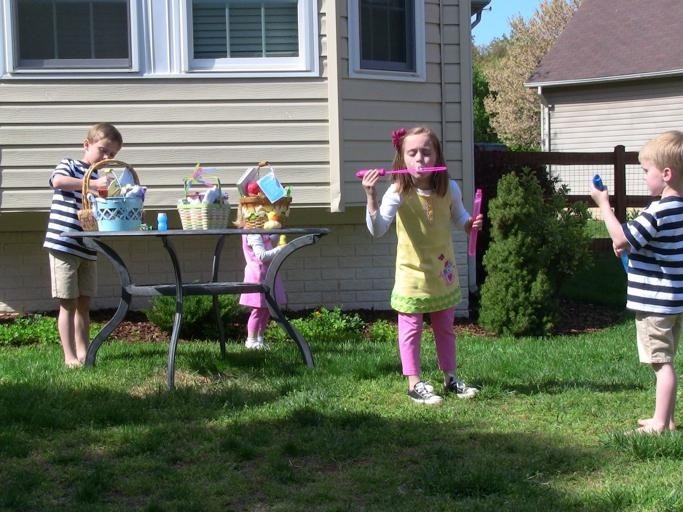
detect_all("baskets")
[77,159,144,231]
[177,176,230,230]
[240,163,292,229]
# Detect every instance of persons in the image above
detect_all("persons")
[229,203,289,353]
[43,122,124,372]
[362,128,485,405]
[589,130,681,438]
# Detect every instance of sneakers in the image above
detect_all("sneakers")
[446,376,476,399]
[245,340,267,350]
[408,381,443,404]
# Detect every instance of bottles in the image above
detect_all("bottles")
[468,189,483,256]
[157,212,168,231]
[593,174,629,276]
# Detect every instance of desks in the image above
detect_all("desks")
[58,227,330,394]
